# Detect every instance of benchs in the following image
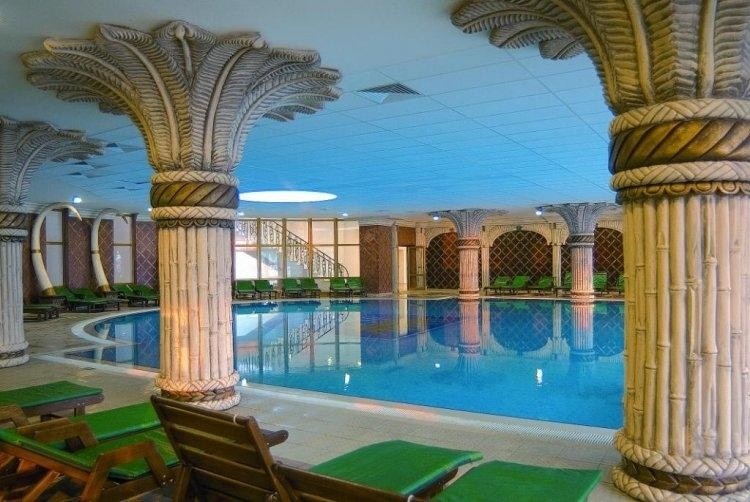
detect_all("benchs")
[425,458,606,501]
[1,377,105,424]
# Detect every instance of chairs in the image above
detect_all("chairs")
[21,283,159,321]
[151,394,481,502]
[2,402,162,479]
[232,276,368,301]
[2,421,289,500]
[484,272,624,298]
